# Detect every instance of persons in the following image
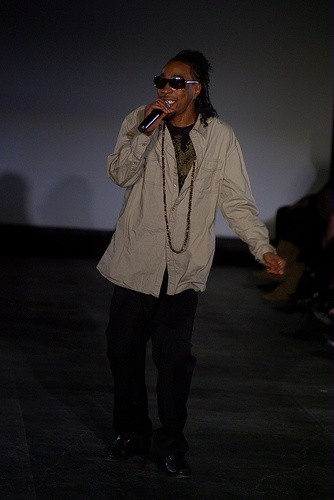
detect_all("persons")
[98,49,285,478]
[260,184,334,347]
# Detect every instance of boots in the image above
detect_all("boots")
[261,262,305,302]
[250,240,299,283]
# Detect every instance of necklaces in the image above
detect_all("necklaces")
[161,121,195,253]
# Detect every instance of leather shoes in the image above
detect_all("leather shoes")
[150,438,193,479]
[102,430,139,462]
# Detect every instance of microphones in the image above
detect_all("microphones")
[138,100,171,133]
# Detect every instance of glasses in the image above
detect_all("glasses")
[153,74,200,89]
